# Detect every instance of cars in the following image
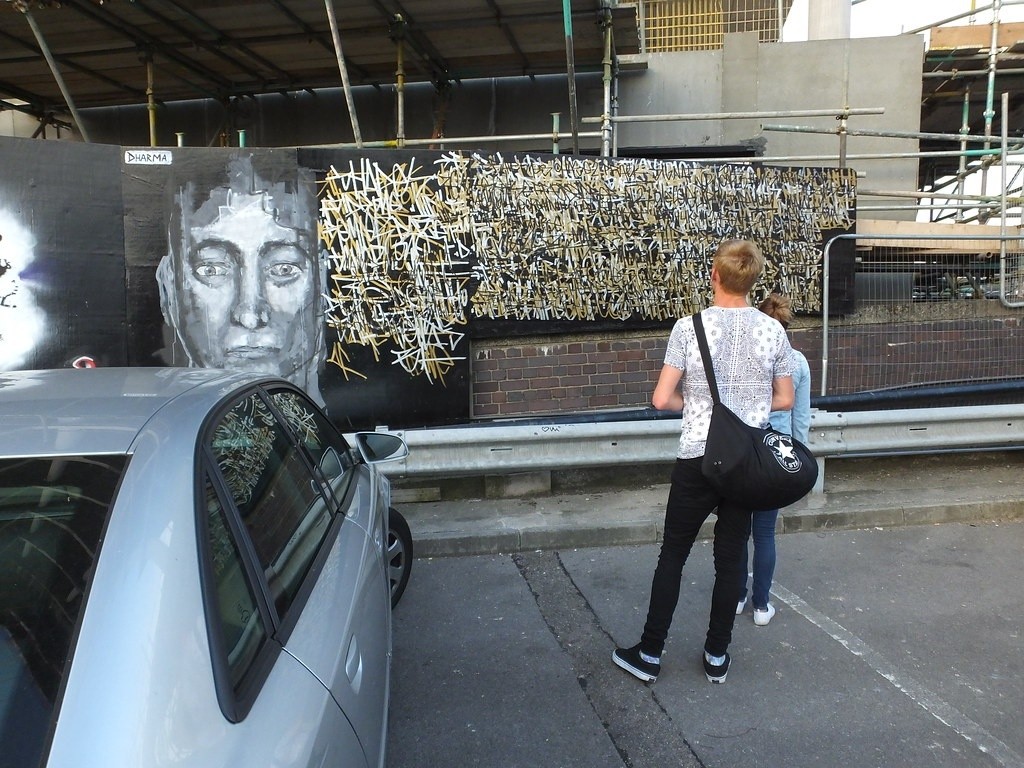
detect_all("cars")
[0,367,416,768]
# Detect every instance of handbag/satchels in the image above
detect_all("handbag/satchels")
[702,404,818,512]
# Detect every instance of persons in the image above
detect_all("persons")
[736,296,810,626]
[612,240,793,685]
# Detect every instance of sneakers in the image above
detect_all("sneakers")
[736,597,749,615]
[611,643,661,684]
[753,601,775,626]
[702,648,730,684]
